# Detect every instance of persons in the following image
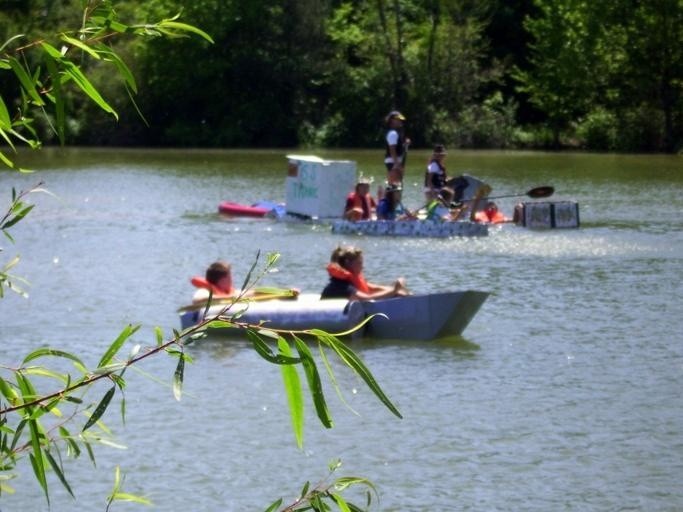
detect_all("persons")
[320,247,413,300]
[344,108,511,226]
[192,262,256,304]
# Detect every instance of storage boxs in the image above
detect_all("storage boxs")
[284,153,359,220]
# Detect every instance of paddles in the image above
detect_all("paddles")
[176,292,294,312]
[459,186,554,201]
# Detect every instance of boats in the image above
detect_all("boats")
[174,267,499,342]
[224,202,488,239]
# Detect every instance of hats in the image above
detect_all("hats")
[389,110,406,122]
[432,144,448,156]
